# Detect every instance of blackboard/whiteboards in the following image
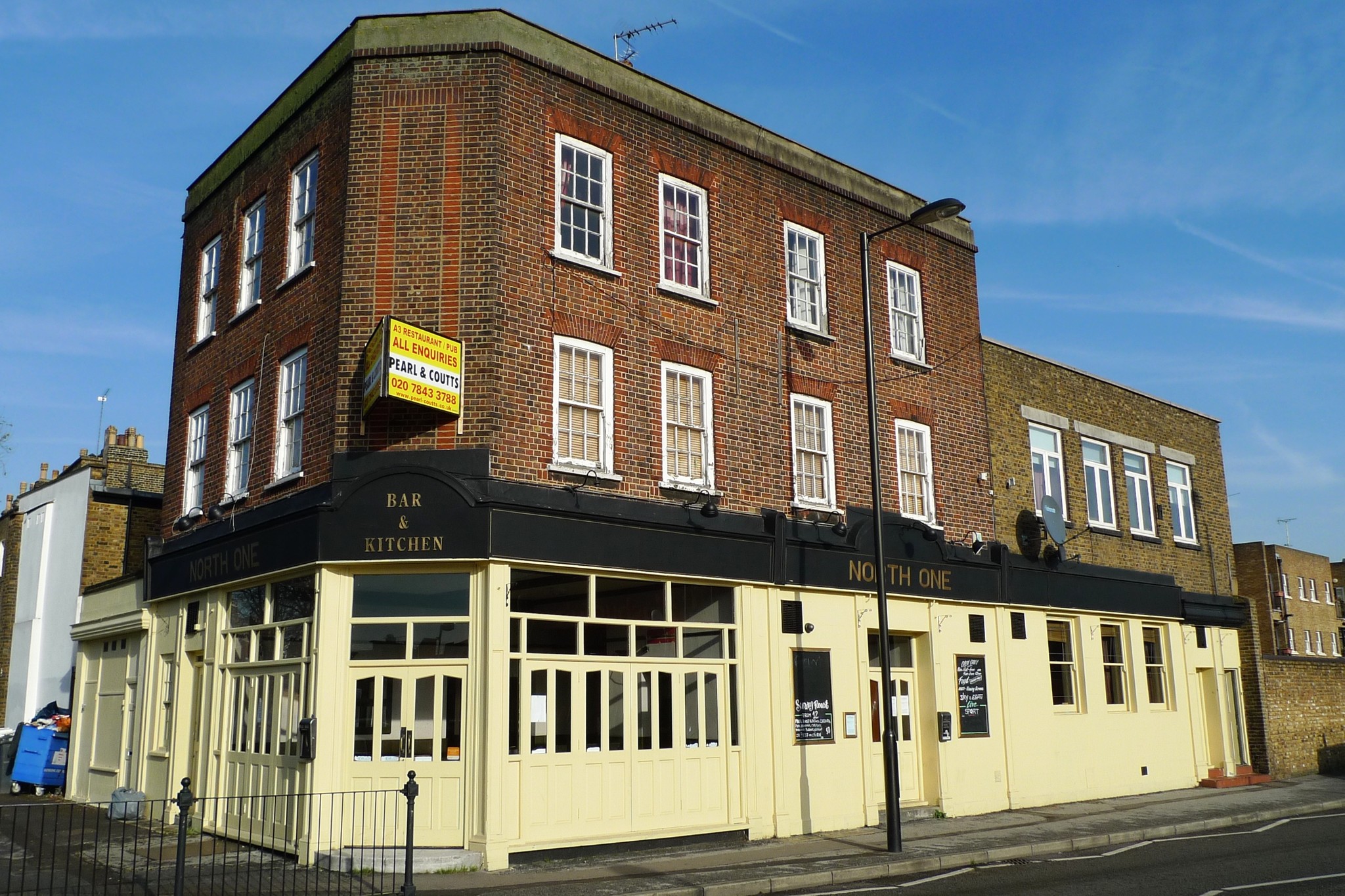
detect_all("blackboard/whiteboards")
[789,647,837,745]
[953,654,990,738]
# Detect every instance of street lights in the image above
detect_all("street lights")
[859,195,967,851]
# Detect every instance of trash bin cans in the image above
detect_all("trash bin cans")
[10,724,71,797]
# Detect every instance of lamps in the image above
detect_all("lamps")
[978,473,988,481]
[1053,551,1082,564]
[683,490,719,517]
[814,511,847,537]
[566,470,600,493]
[178,507,225,531]
[209,493,255,520]
[901,523,938,542]
[951,530,984,555]
[1005,477,1015,486]
